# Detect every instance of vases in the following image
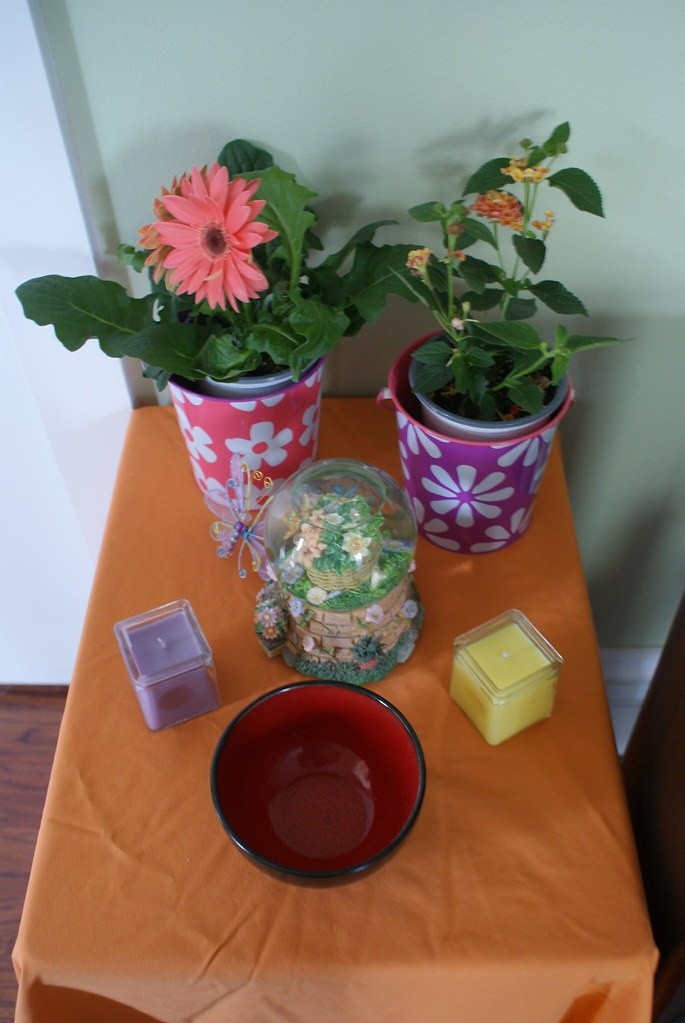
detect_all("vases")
[196,356,322,397]
[409,332,570,442]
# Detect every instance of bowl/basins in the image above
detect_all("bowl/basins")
[209,679,428,888]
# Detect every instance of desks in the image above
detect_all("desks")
[10,398,664,1023]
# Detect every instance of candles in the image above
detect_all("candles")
[450,622,556,746]
[127,612,220,732]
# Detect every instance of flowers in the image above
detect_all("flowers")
[387,119,637,424]
[249,482,417,670]
[13,139,460,391]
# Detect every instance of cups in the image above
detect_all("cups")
[449,608,562,746]
[113,598,220,732]
